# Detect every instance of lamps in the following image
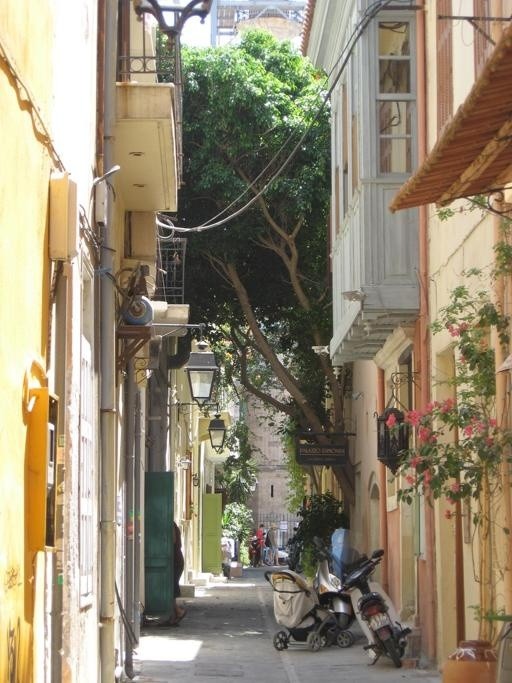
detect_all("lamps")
[192,473,200,486]
[151,322,219,411]
[178,402,227,452]
[376,372,421,475]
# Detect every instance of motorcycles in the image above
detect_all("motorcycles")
[285,526,304,573]
[335,548,412,667]
[311,527,372,630]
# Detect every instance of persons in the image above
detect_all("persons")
[268,522,280,565]
[256,524,267,567]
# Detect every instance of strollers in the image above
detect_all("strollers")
[265,566,356,653]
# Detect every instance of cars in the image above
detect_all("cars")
[248,536,289,566]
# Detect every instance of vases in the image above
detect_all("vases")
[443,640,497,683]
